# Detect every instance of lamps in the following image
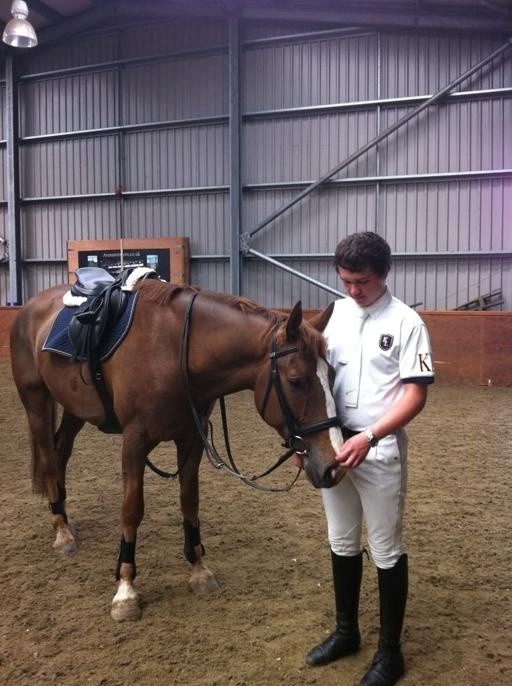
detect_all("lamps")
[2,0,40,48]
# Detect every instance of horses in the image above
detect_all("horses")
[9,277,350,623]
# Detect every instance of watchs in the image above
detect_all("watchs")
[362,427,379,449]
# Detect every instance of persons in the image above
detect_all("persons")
[291,230,436,684]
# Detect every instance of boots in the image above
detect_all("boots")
[304,551,366,666]
[356,555,408,686]
